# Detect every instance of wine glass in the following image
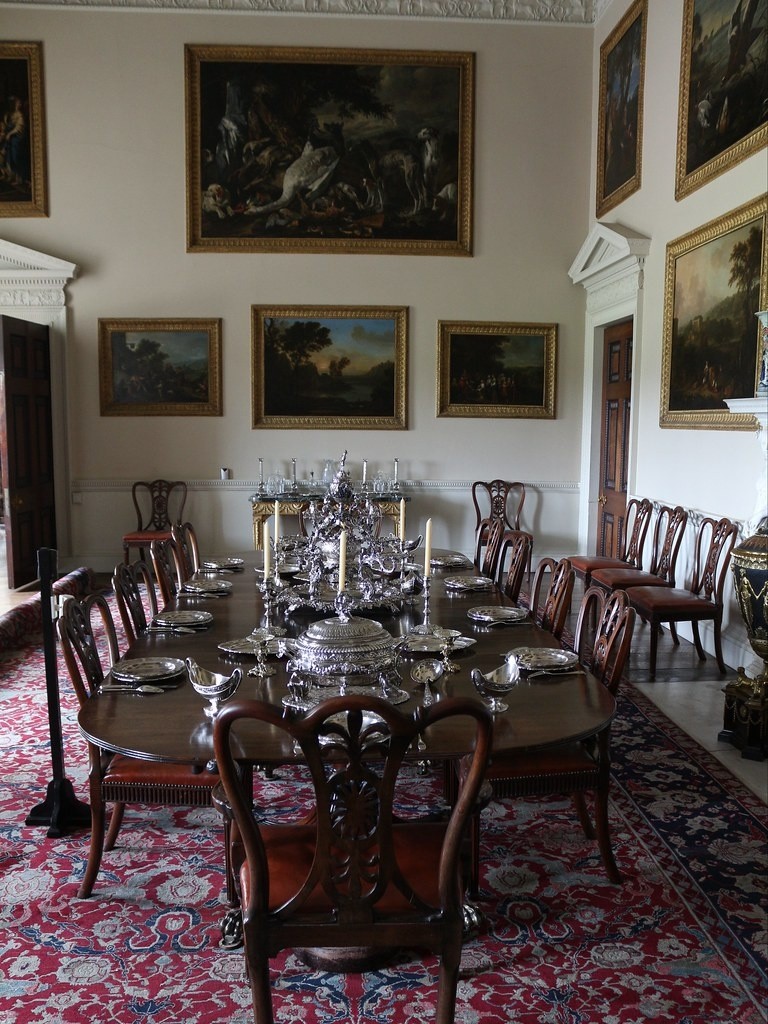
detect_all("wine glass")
[245,634,276,678]
[184,657,244,715]
[400,570,424,605]
[433,628,463,674]
[470,656,520,713]
[403,535,423,554]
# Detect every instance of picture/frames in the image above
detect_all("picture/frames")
[435,318,559,419]
[184,41,477,257]
[0,39,48,219]
[594,1,648,224]
[250,303,411,432]
[674,0,768,201]
[659,194,768,430]
[96,317,224,417]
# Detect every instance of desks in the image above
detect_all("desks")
[247,490,412,550]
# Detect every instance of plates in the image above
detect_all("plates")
[254,563,299,573]
[430,554,468,567]
[203,557,245,568]
[466,605,526,622]
[504,647,580,671]
[443,575,494,590]
[111,656,187,682]
[390,635,477,652]
[182,579,233,592]
[152,610,214,627]
[217,637,298,654]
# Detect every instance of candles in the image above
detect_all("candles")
[394,457,399,482]
[400,496,405,540]
[337,529,346,590]
[362,459,367,483]
[291,458,297,483]
[262,519,271,579]
[274,498,280,543]
[425,517,431,576]
[259,456,264,483]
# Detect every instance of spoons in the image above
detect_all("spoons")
[199,569,234,574]
[175,593,219,599]
[146,626,196,634]
[97,684,164,694]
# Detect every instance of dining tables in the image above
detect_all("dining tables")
[77,541,622,951]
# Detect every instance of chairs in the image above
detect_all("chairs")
[54,479,740,1024]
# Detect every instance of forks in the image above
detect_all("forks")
[459,588,492,593]
[527,670,587,679]
[486,621,533,628]
